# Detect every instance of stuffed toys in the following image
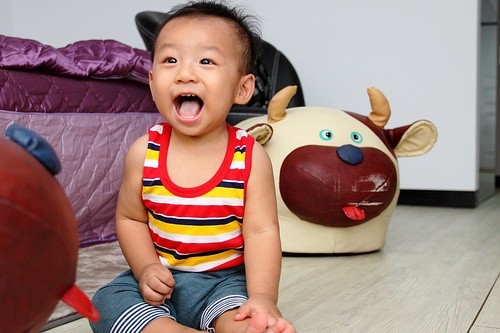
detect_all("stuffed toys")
[0,124,100,333]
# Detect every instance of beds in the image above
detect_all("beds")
[0,69,166,248]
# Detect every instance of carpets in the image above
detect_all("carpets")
[38,241,131,333]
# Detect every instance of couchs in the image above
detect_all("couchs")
[135,11,305,126]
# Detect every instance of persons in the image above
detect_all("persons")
[88,0,298,333]
